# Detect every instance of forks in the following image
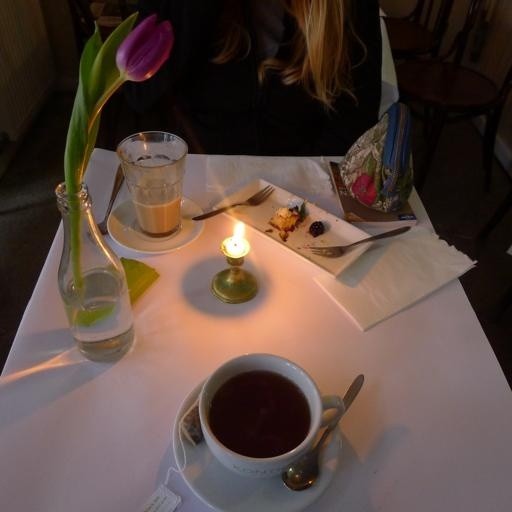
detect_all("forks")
[309,226,415,258]
[192,184,274,220]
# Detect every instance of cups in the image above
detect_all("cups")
[195,352,346,486]
[117,130,187,239]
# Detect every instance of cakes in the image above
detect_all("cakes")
[268,205,301,232]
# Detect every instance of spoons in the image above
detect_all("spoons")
[83,155,130,240]
[276,369,365,488]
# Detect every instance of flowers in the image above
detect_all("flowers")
[57,8,176,316]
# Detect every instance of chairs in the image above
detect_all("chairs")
[383,2,512,203]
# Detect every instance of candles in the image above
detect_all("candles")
[233,224,246,255]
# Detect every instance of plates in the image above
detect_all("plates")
[100,198,204,258]
[171,379,346,512]
[211,178,377,278]
[328,160,418,227]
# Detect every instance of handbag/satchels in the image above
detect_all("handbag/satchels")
[339,101,413,213]
